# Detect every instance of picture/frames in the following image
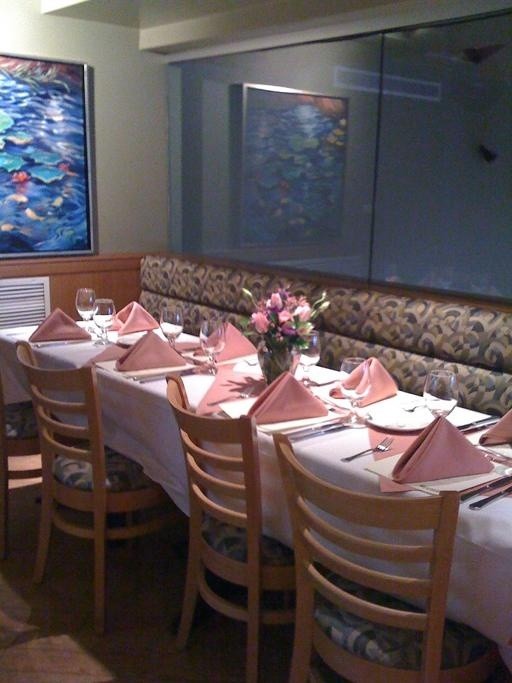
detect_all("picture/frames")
[226,79,351,252]
[1,51,95,259]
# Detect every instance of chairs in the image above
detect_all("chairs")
[155,368,329,680]
[0,365,92,566]
[267,424,508,681]
[13,338,195,642]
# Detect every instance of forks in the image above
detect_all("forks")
[340,435,395,466]
[240,387,255,400]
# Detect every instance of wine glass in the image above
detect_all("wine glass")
[336,355,374,429]
[423,368,460,421]
[75,285,323,387]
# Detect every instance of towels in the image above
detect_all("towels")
[26,305,93,345]
[388,412,497,485]
[477,405,512,447]
[242,369,329,428]
[339,355,399,409]
[199,319,257,363]
[114,327,189,374]
[104,300,161,336]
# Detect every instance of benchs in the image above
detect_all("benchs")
[134,246,511,431]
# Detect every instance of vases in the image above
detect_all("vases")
[252,330,309,388]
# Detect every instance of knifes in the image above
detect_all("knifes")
[288,422,352,442]
[461,476,512,511]
[457,414,500,435]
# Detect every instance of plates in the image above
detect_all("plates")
[365,403,434,432]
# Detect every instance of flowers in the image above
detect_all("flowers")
[230,278,334,396]
